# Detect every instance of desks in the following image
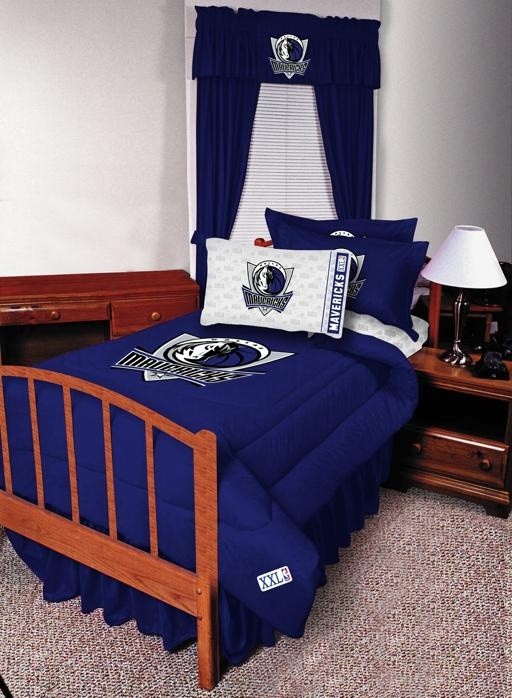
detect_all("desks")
[1,270,200,340]
[440,303,505,344]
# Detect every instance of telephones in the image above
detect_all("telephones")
[474,343,512,380]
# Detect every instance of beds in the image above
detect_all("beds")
[1,238,442,691]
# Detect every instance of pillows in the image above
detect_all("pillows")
[274,218,429,342]
[265,208,417,244]
[199,237,352,340]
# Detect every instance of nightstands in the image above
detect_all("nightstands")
[394,347,512,519]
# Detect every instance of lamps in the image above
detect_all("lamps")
[420,225,508,365]
